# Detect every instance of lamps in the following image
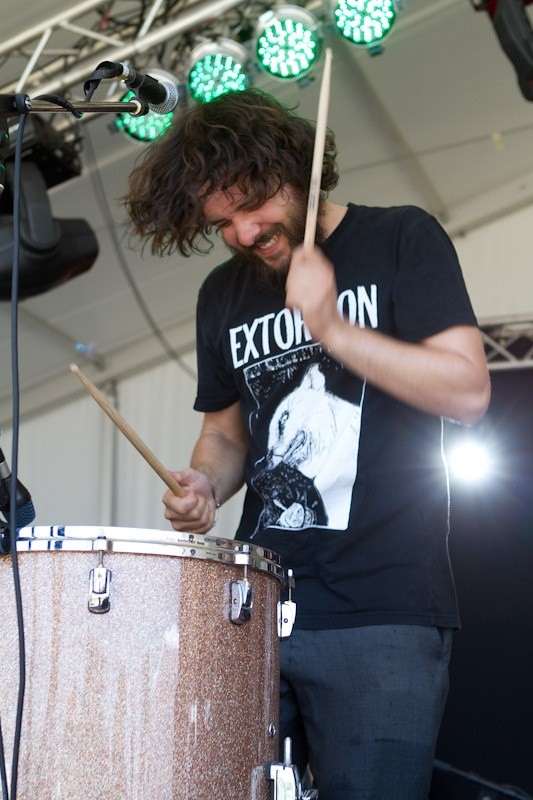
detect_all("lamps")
[0,109,100,306]
[328,0,399,57]
[181,26,253,108]
[251,0,335,89]
[115,64,187,147]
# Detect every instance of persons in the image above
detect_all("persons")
[118,88,491,800]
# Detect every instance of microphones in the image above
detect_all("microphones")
[0,448,36,528]
[115,63,179,114]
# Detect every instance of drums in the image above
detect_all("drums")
[0,522,293,800]
